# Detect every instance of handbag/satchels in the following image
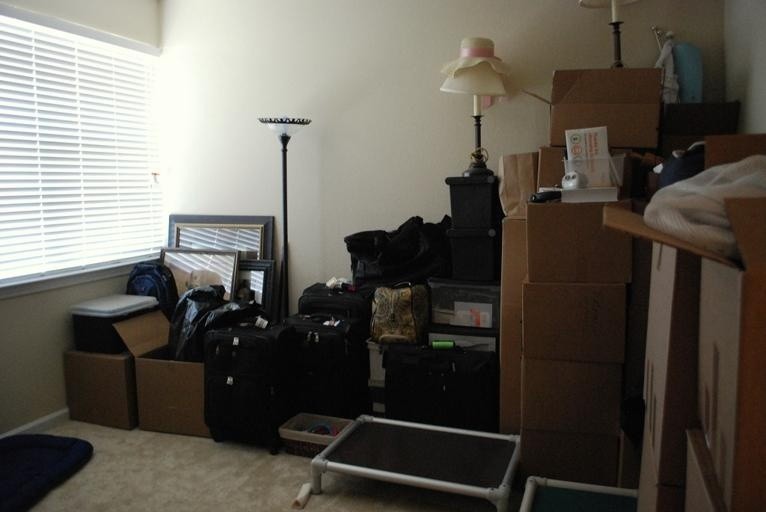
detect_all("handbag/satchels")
[370,281,430,345]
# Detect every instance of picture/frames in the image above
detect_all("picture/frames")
[233,257,277,327]
[160,245,239,313]
[167,211,276,264]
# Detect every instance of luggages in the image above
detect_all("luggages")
[203,283,370,455]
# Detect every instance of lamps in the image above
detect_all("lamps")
[257,110,312,322]
[577,0,651,71]
[436,34,510,177]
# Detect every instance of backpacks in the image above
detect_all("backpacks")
[126,261,179,322]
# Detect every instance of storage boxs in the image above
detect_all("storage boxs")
[442,226,502,282]
[113,306,217,441]
[444,172,497,226]
[519,428,622,511]
[377,344,502,433]
[60,348,142,434]
[429,278,499,328]
[422,329,496,347]
[501,216,528,439]
[521,274,625,358]
[68,292,159,352]
[534,144,637,201]
[525,199,633,286]
[601,97,764,510]
[548,66,666,151]
[517,357,624,438]
